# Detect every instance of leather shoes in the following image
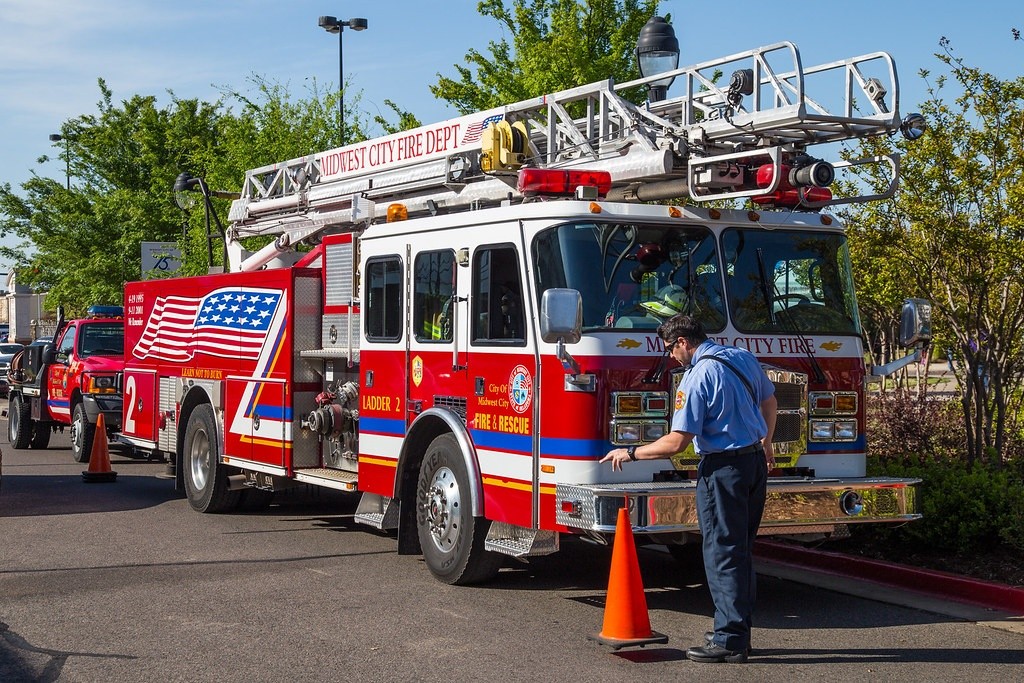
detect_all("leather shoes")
[703,631,753,652]
[686,642,748,664]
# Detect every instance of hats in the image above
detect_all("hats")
[639,285,690,318]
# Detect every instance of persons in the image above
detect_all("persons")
[598,314,776,664]
[0,334,9,343]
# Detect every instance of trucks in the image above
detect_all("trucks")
[0,304,125,463]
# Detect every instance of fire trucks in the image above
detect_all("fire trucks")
[110,40,936,588]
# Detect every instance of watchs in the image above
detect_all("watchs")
[627,446,638,461]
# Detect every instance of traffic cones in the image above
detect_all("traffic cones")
[81,412,120,484]
[585,508,670,650]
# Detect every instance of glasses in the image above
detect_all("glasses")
[665,339,677,353]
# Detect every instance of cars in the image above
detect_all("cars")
[0,342,26,398]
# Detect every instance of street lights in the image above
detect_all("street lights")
[49,134,79,191]
[317,15,369,148]
